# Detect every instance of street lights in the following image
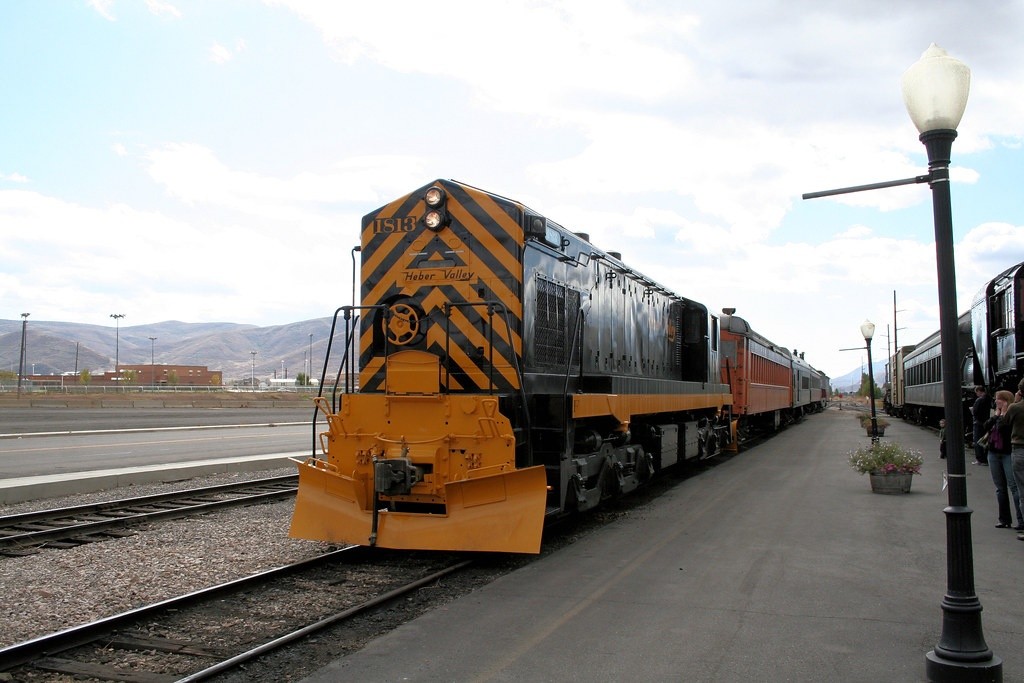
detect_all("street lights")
[797,41,1004,683]
[838,317,880,446]
[250,350,258,388]
[281,360,284,379]
[110,313,124,393]
[147,337,157,392]
[17,312,30,398]
[308,333,314,380]
[305,358,308,389]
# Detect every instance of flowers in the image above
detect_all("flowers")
[855,410,872,418]
[844,437,925,476]
[862,417,890,429]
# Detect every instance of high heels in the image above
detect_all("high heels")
[995,522,1012,528]
[1013,525,1024,530]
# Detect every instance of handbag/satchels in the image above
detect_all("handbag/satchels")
[974,431,989,457]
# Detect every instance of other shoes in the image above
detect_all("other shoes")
[971,460,980,465]
[1017,535,1024,541]
[980,463,988,466]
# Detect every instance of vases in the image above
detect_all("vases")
[860,418,864,427]
[868,471,913,494]
[866,428,885,437]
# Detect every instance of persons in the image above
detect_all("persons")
[983,378,1024,541]
[972,385,991,466]
[961,387,976,436]
[937,419,947,459]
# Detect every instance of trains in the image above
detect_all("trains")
[883,260,1024,430]
[288,177,830,554]
